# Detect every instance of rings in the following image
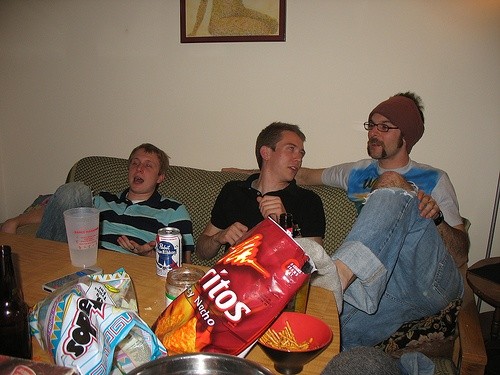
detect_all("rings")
[130,247,135,250]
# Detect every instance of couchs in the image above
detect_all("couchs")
[10,155,487,374]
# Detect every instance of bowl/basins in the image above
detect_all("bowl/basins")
[256,311,334,375]
[125,352,274,375]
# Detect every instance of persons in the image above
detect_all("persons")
[221,89,465,348]
[196,123,326,260]
[0,143,195,263]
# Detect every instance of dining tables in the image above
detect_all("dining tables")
[0,233,342,375]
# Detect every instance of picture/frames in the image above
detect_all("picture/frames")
[180,0,286,44]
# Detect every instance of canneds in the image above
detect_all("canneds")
[155,227,182,277]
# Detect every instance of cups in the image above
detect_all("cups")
[63,207,100,267]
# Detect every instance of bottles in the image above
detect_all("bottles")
[279,213,295,239]
[0,245,32,361]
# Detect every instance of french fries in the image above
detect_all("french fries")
[260,320,313,351]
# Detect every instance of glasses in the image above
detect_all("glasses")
[363,122,399,132]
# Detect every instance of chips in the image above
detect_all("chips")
[154,313,196,355]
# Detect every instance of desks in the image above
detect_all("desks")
[465,257,500,340]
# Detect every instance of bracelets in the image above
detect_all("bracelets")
[434,210,444,226]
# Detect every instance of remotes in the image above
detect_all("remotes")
[41,265,103,293]
